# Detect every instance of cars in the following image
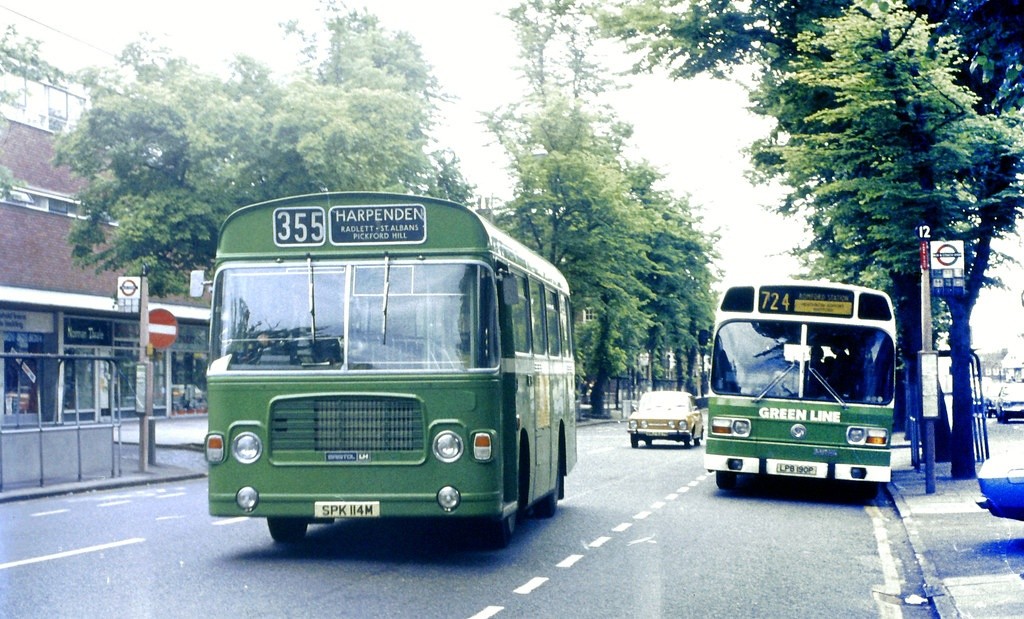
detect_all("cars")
[971,377,1024,424]
[627,391,705,449]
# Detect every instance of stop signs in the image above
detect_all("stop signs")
[148,307,180,349]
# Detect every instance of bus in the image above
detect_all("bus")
[189,188,577,549]
[698,278,904,504]
